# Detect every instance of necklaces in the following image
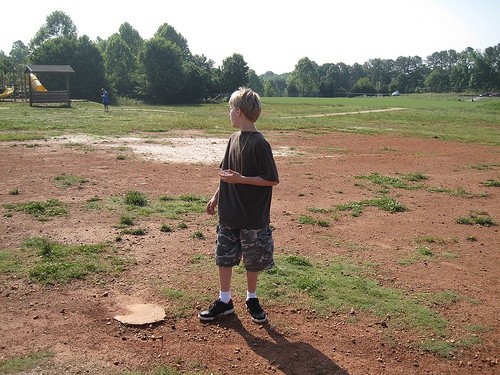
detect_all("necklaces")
[238,127,256,175]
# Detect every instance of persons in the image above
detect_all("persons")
[100,88,109,112]
[198,87,281,324]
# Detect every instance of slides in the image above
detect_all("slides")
[0,88,14,99]
[25,71,48,92]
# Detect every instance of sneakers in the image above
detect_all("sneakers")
[198,298,235,320]
[245,298,266,322]
